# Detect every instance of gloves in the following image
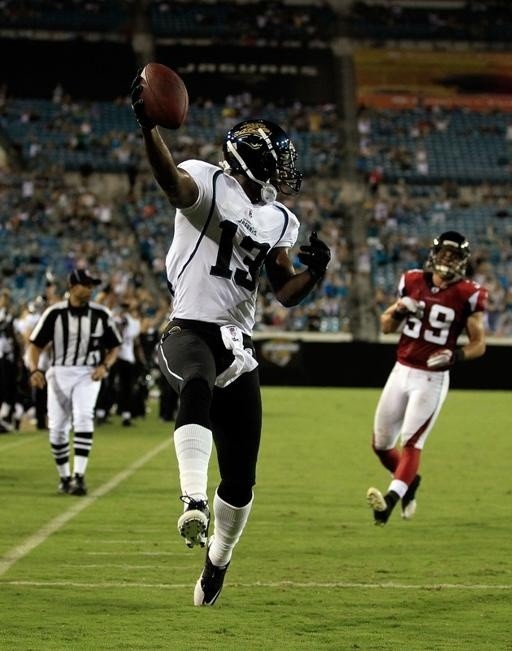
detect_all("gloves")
[393,297,417,321]
[298,230,330,278]
[129,68,154,129]
[427,349,458,367]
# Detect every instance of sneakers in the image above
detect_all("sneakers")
[194,534,232,606]
[367,475,420,526]
[178,499,211,548]
[59,473,87,494]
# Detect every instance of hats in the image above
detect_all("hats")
[69,269,101,286]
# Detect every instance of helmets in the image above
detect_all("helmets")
[223,120,301,196]
[433,233,469,280]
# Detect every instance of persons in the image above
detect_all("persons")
[1,1,511,430]
[365,231,486,528]
[26,269,123,496]
[128,73,330,607]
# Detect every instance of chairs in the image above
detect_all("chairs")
[2,91,512,333]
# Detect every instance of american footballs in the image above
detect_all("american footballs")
[138,63,187,130]
